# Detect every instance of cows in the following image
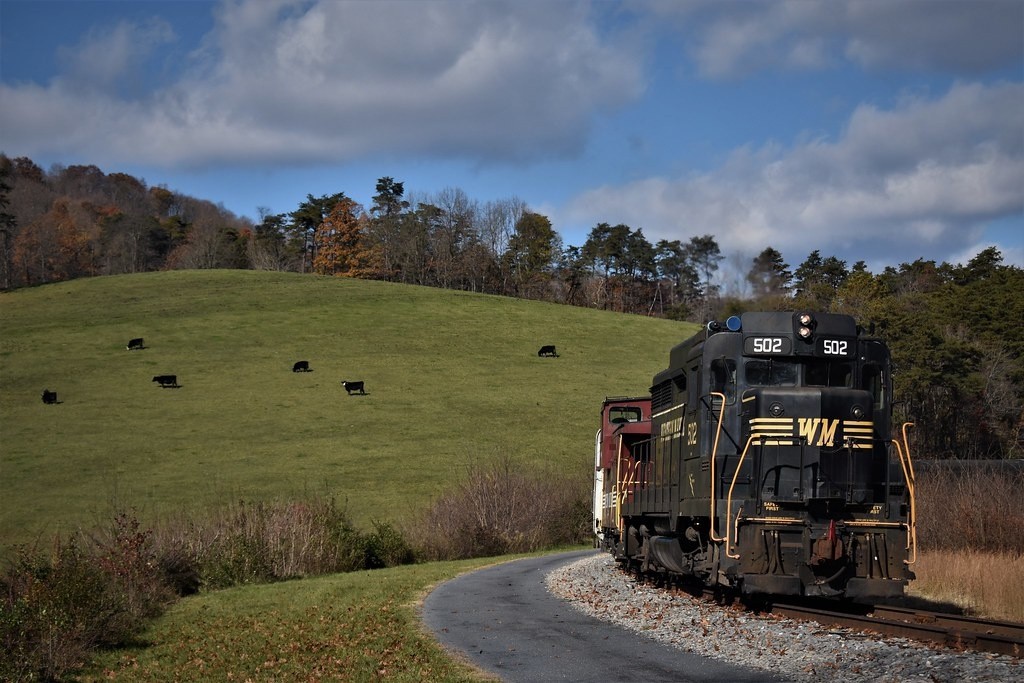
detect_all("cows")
[293,361,308,372]
[126,338,145,351]
[538,346,556,357]
[42,389,57,404]
[152,375,177,388]
[341,381,364,395]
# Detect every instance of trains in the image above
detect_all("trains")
[592,311,918,603]
